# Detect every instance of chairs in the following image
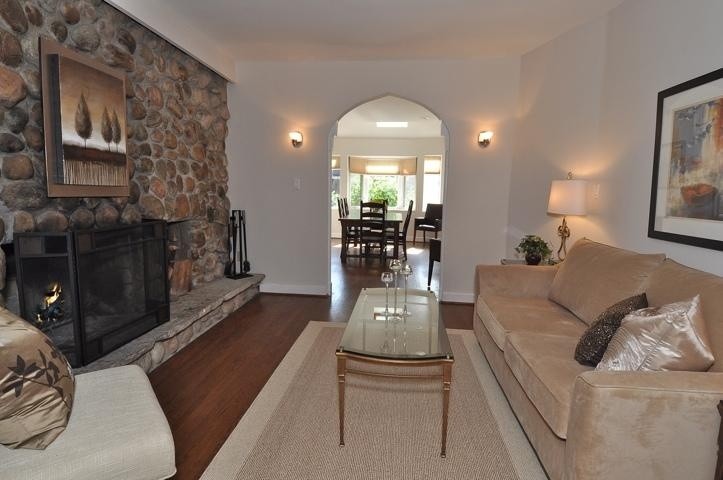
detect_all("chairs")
[337,196,444,291]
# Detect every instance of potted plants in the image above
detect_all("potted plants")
[514,234,551,265]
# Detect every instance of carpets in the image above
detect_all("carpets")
[195,321,552,480]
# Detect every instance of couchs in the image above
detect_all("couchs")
[473,237,722,480]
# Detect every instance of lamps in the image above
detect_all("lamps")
[545,171,592,262]
[289,130,302,148]
[477,129,493,146]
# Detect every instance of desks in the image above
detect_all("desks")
[501,257,556,265]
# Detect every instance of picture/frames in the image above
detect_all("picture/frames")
[647,67,723,254]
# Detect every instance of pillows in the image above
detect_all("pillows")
[0,307,76,451]
[572,291,647,366]
[594,294,714,371]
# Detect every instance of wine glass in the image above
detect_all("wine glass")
[399,264,412,315]
[380,272,392,316]
[389,259,401,317]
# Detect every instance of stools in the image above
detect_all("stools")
[0,363,179,480]
